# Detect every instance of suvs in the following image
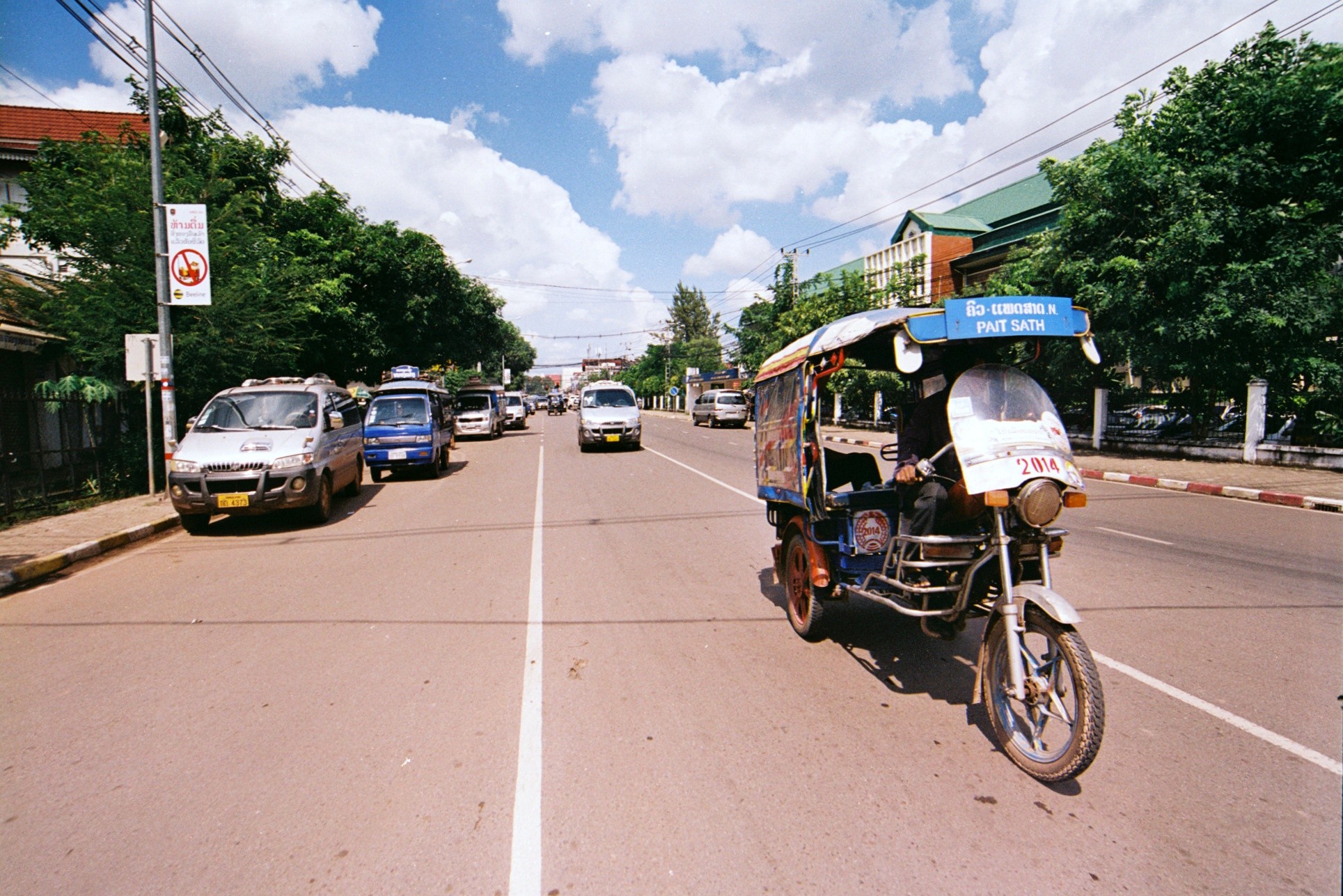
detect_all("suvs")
[167,376,366,534]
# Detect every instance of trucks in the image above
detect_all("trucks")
[575,379,644,453]
[451,384,507,441]
[501,390,579,430]
[362,365,458,484]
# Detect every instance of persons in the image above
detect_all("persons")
[893,350,998,589]
[824,447,883,492]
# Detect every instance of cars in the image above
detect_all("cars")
[1110,402,1299,446]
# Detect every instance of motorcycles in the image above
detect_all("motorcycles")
[753,295,1106,785]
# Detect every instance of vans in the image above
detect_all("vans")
[692,388,749,429]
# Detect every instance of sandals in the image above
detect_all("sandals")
[900,564,928,589]
